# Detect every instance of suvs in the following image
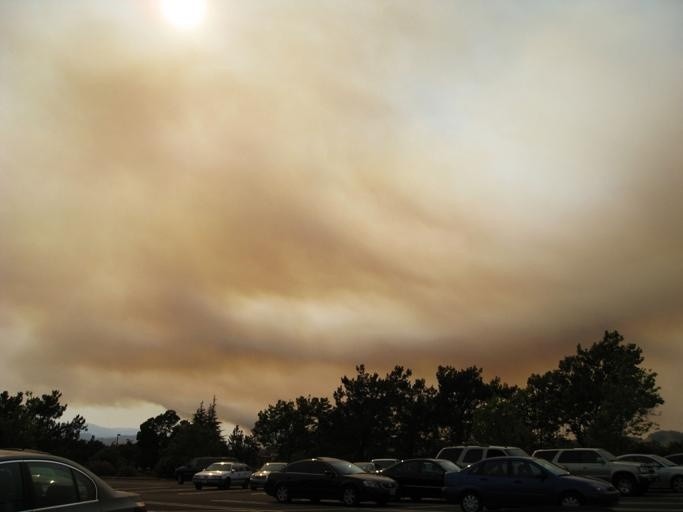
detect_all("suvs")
[191,461,254,492]
[612,453,683,496]
[173,455,239,485]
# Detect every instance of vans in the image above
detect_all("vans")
[435,444,528,469]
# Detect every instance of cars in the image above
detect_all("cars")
[530,446,658,494]
[0,446,148,511]
[440,454,625,510]
[266,455,399,508]
[249,462,288,492]
[379,457,465,509]
